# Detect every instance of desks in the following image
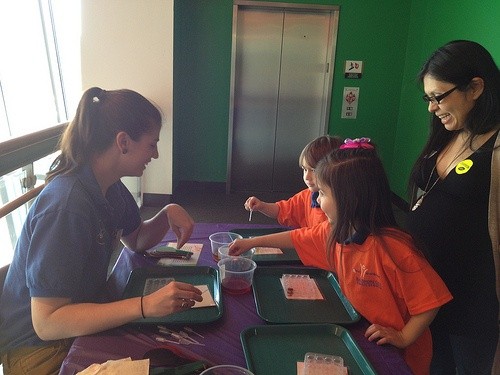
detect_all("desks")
[57,221,414,375]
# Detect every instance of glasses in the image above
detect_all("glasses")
[422,84,463,105]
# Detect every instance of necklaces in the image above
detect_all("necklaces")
[412,136,477,211]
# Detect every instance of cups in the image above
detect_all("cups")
[199,364,255,375]
[220,244,256,271]
[217,257,257,295]
[209,231,244,262]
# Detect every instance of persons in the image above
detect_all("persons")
[0,87,202,375]
[244,135,345,229]
[411,40,500,375]
[229,137,454,375]
[141,296,144,318]
